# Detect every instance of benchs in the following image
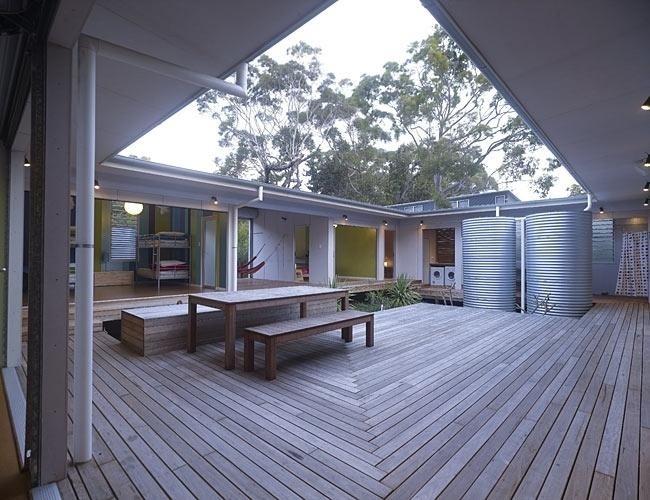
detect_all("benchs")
[243,310,374,381]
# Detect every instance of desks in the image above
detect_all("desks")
[186,285,351,370]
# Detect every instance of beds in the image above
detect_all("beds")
[136,230,191,291]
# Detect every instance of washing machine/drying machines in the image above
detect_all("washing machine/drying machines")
[444,266,455,287]
[430,266,445,286]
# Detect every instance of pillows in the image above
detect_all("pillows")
[155,232,186,238]
[155,260,186,267]
